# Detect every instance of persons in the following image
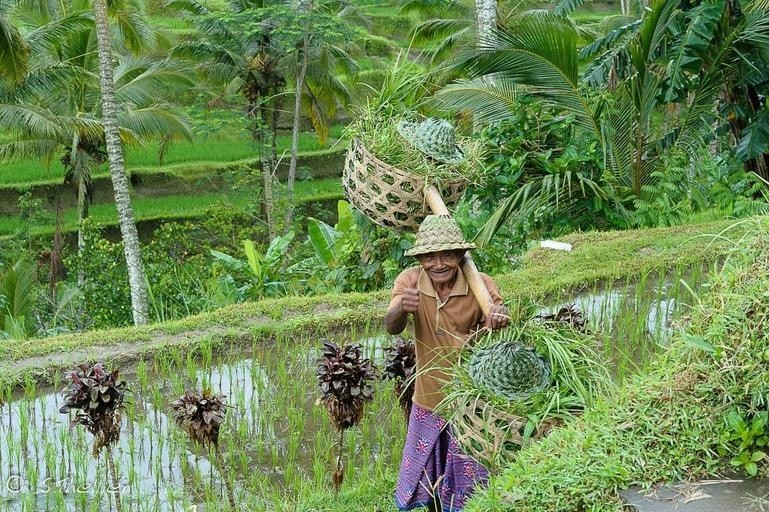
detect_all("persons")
[386,214,511,511]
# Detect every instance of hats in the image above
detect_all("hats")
[404,214,476,257]
[466,337,552,403]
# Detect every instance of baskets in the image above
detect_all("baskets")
[448,315,584,472]
[340,137,468,233]
